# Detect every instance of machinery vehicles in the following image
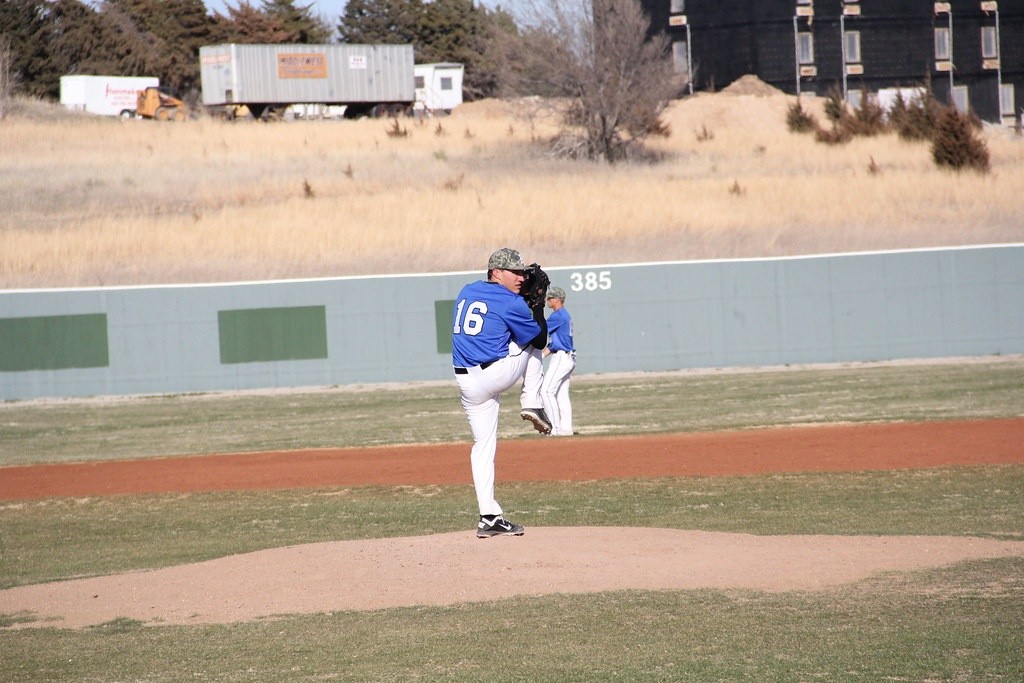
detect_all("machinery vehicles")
[135,86,259,123]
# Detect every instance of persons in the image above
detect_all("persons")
[539,287,577,437]
[452,247,553,538]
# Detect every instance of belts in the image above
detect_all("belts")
[455,361,496,374]
[551,351,567,353]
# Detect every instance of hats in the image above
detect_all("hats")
[488,248,535,270]
[545,287,565,302]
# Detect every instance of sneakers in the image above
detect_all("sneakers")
[520,408,552,434]
[476,516,524,537]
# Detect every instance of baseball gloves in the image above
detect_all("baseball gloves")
[520,262,552,308]
